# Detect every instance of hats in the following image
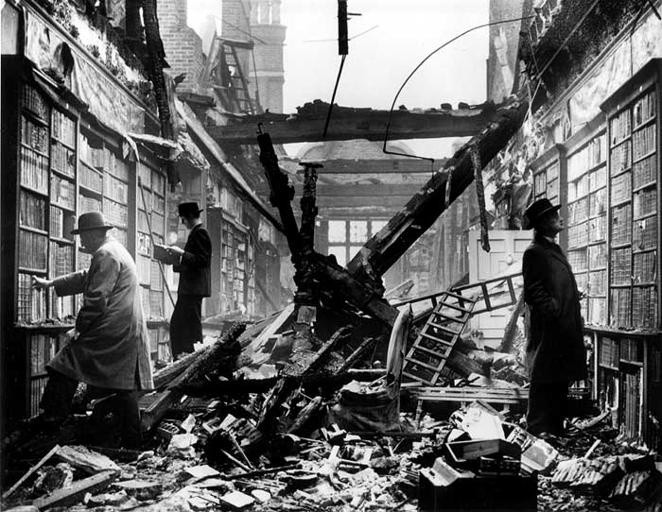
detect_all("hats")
[522,199,561,230]
[177,202,204,216]
[70,212,113,233]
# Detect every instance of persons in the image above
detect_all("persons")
[155,202,215,367]
[518,197,587,437]
[26,211,159,448]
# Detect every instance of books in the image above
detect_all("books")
[20,82,164,412]
[533,93,662,451]
[216,216,248,313]
[152,242,181,268]
